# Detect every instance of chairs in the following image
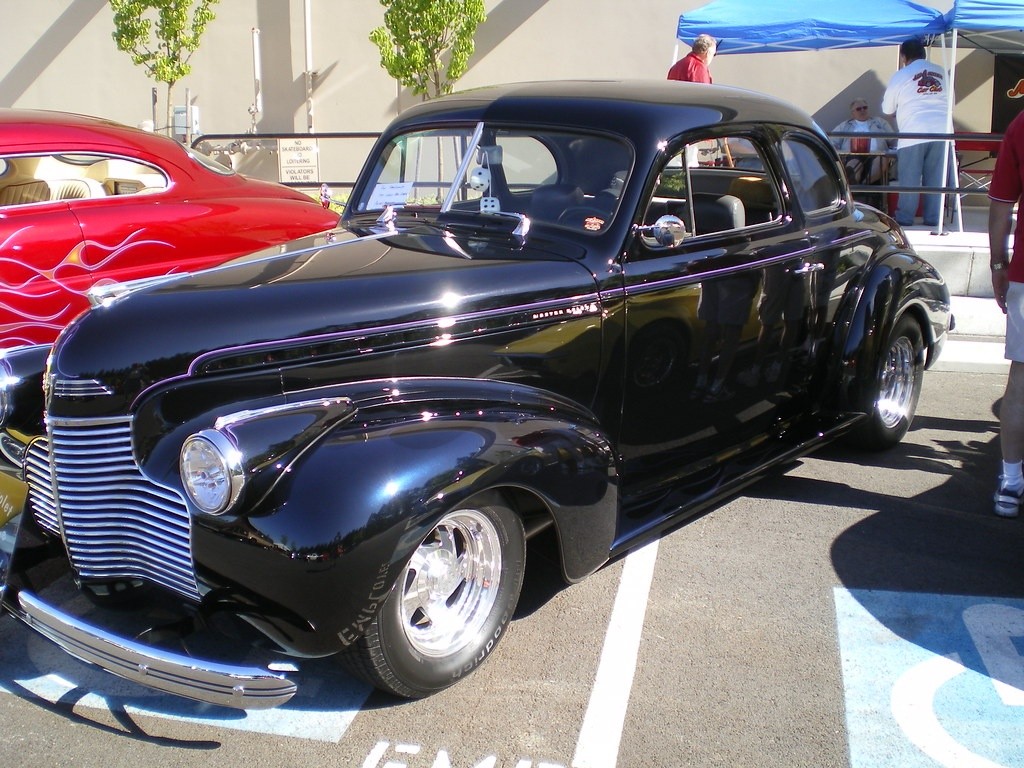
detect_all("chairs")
[531,175,778,235]
[0,176,107,206]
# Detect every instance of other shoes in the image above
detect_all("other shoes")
[891,217,911,226]
[924,221,936,226]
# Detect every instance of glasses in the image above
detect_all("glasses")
[853,105,870,112]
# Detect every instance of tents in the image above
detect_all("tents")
[670,0,1024,235]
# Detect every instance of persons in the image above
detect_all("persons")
[989,109,1024,517]
[827,98,898,198]
[667,35,716,168]
[881,38,955,227]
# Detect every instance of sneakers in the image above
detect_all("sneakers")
[993,484,1024,517]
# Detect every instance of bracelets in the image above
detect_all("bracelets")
[990,261,1009,270]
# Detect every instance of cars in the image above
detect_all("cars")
[0,107,342,352]
[0,79,955,710]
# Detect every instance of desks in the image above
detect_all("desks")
[837,150,898,215]
